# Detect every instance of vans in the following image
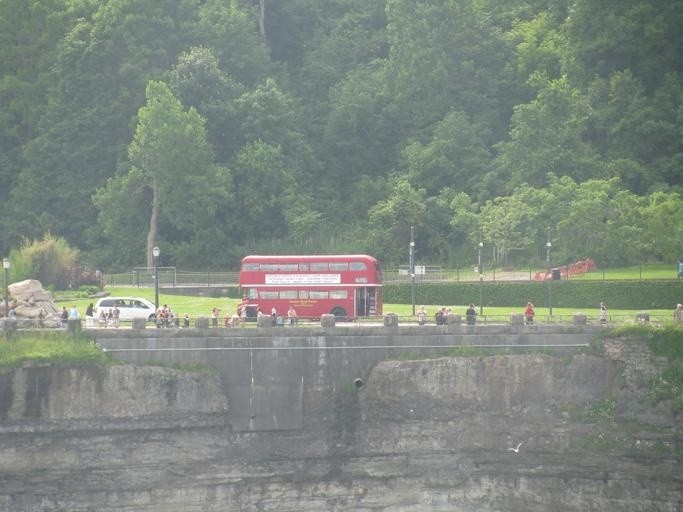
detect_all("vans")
[93,297,160,322]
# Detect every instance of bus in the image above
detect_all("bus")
[238,254,383,321]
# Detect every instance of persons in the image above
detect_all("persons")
[212,304,248,326]
[525,303,535,325]
[677,260,682,280]
[673,304,681,326]
[155,305,189,327]
[675,258,679,278]
[256,305,297,325]
[600,304,607,325]
[0,296,34,318]
[60,303,120,327]
[417,305,427,325]
[437,308,451,325]
[467,304,477,325]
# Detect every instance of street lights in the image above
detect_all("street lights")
[544,227,555,316]
[478,227,486,317]
[1,257,11,334]
[152,246,162,313]
[409,224,418,316]
